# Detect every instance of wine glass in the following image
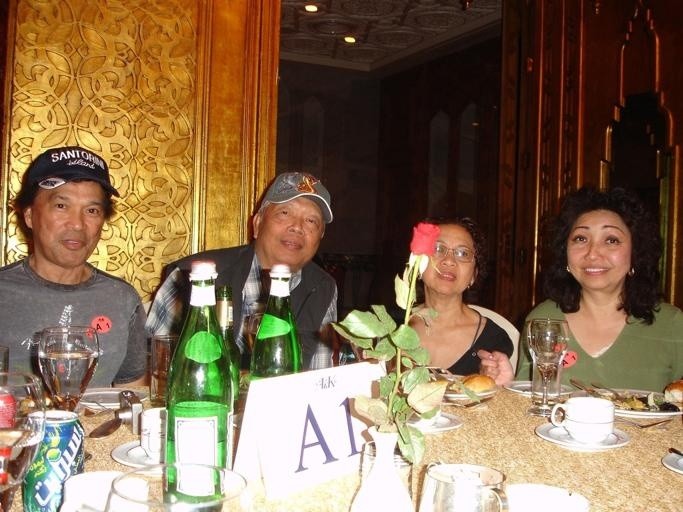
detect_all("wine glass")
[244,312,295,367]
[104,461,250,512]
[0,371,48,511]
[525,318,571,419]
[37,325,100,461]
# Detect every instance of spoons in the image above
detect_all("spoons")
[622,415,674,431]
[85,417,122,439]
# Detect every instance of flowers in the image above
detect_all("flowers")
[330,222,481,465]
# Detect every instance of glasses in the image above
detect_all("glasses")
[433,243,476,264]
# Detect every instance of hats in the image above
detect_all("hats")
[23,147,120,195]
[261,171,333,223]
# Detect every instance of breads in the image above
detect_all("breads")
[456,375,496,392]
[664,380,682,403]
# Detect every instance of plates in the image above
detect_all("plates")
[407,412,465,433]
[111,439,150,468]
[78,387,150,410]
[661,449,683,475]
[533,421,632,452]
[507,482,593,512]
[568,388,683,419]
[503,380,575,397]
[444,374,498,401]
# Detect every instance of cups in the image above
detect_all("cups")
[140,407,166,462]
[361,441,413,499]
[150,333,179,408]
[531,351,562,406]
[60,470,149,512]
[550,394,616,442]
[417,462,509,512]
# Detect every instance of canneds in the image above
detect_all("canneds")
[22,410,84,511]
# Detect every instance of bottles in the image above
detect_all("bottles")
[213,285,241,415]
[0,345,18,488]
[164,258,235,506]
[247,262,306,378]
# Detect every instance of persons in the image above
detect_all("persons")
[478,180,683,395]
[0,145,149,390]
[400,215,515,376]
[143,171,340,371]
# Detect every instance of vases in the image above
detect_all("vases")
[350,425,416,512]
